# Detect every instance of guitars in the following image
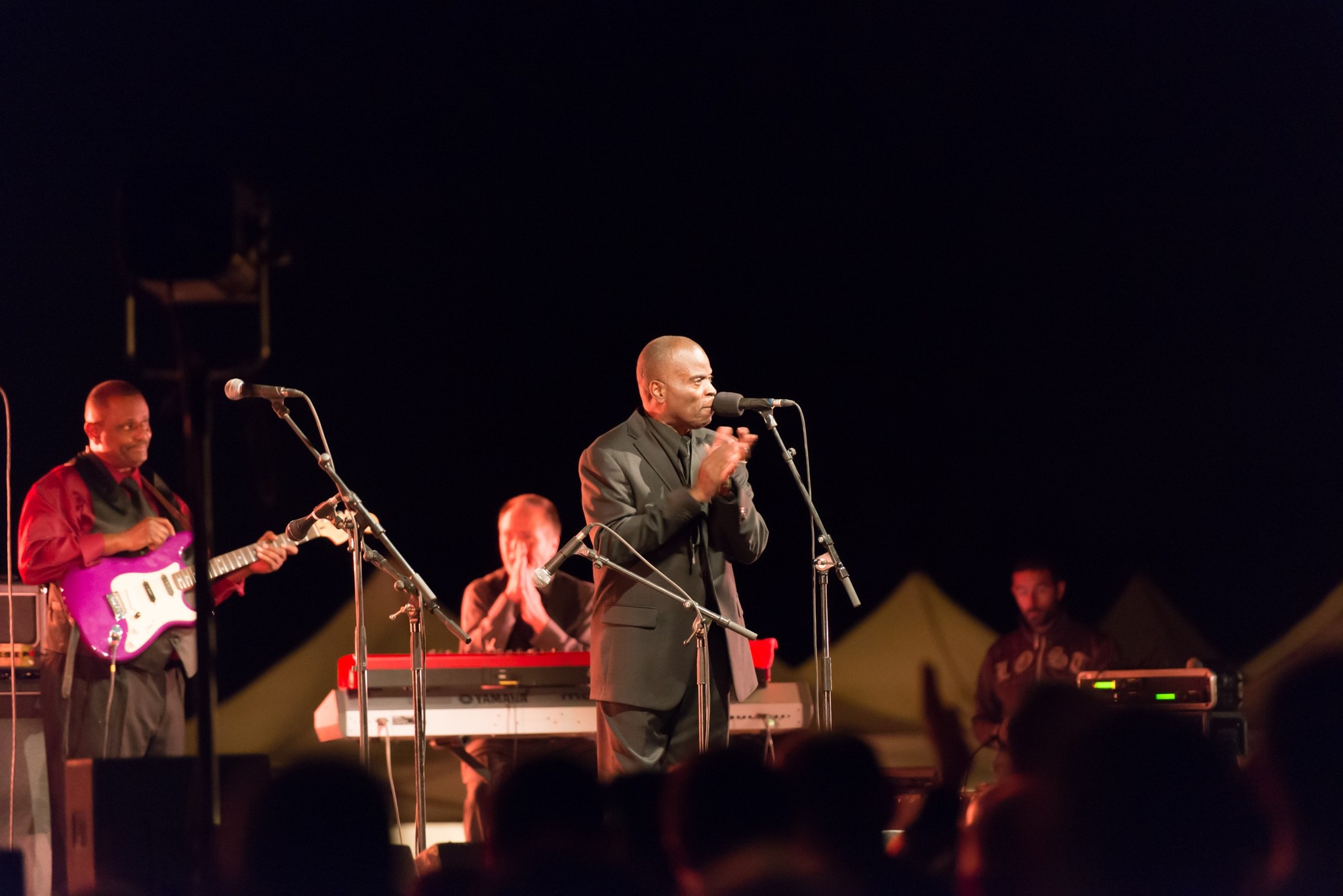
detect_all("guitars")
[57,509,357,666]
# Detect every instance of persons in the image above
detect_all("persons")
[578,335,768,781]
[459,494,595,844]
[970,560,1126,783]
[16,379,299,762]
[234,650,1343,896]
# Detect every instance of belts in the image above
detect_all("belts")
[163,660,182,669]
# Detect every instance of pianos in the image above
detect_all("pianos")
[309,636,819,785]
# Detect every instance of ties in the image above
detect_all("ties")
[678,437,690,484]
[121,477,143,513]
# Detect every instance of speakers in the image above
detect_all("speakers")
[0,585,46,674]
[1,679,269,896]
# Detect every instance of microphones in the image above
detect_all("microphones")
[225,378,303,400]
[285,493,343,541]
[531,524,593,588]
[712,391,795,417]
